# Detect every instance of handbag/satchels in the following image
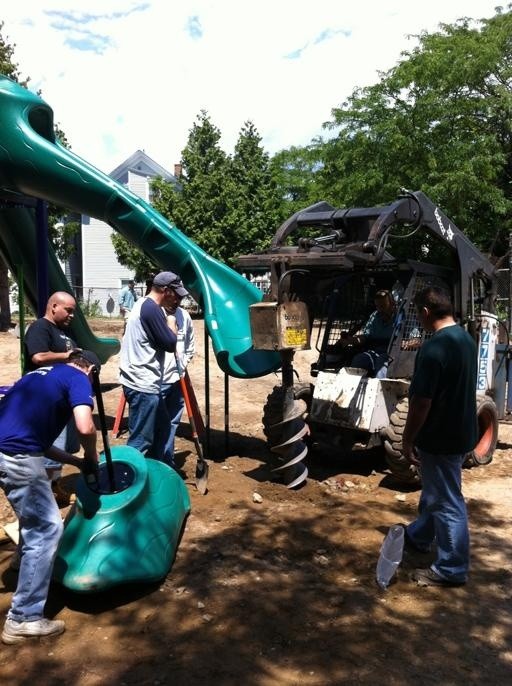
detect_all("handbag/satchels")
[351,350,388,377]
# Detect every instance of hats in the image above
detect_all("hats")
[153,271,190,296]
[69,350,100,374]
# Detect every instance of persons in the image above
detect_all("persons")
[153,279,195,465]
[339,289,421,378]
[399,285,481,588]
[118,270,190,458]
[118,278,138,336]
[22,290,83,482]
[0,348,101,645]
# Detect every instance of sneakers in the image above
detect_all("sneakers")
[10,552,20,570]
[414,569,464,586]
[1,617,65,645]
[396,523,425,554]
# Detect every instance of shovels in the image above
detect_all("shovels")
[174,351,208,496]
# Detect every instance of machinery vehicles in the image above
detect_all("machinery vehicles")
[236,188,512,488]
